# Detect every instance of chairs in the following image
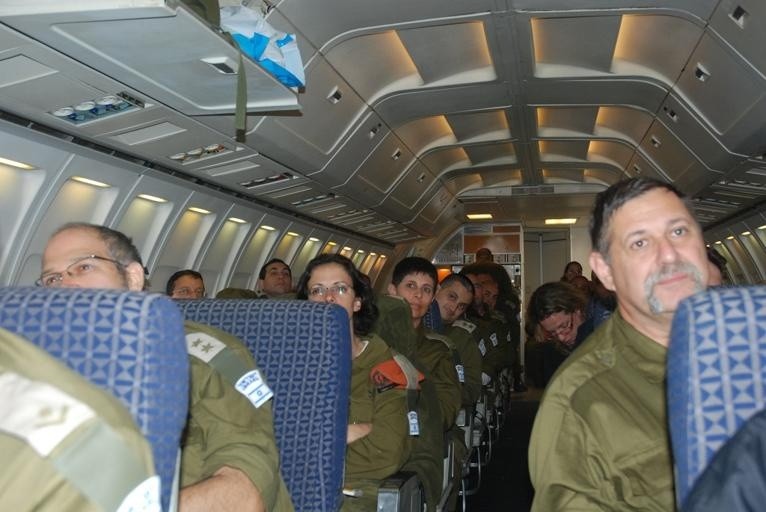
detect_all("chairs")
[589,292,615,331]
[664,280,766,511]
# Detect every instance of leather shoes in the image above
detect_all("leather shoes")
[513,374,526,392]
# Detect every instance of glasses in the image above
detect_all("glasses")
[543,312,573,345]
[308,284,351,297]
[34,255,127,287]
[173,288,204,295]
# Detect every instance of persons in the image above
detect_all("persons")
[432,273,484,510]
[36,221,297,512]
[458,247,615,448]
[166,269,206,301]
[706,245,727,286]
[674,406,766,511]
[0,325,163,511]
[531,178,716,511]
[298,255,411,512]
[255,259,293,297]
[384,258,466,510]
